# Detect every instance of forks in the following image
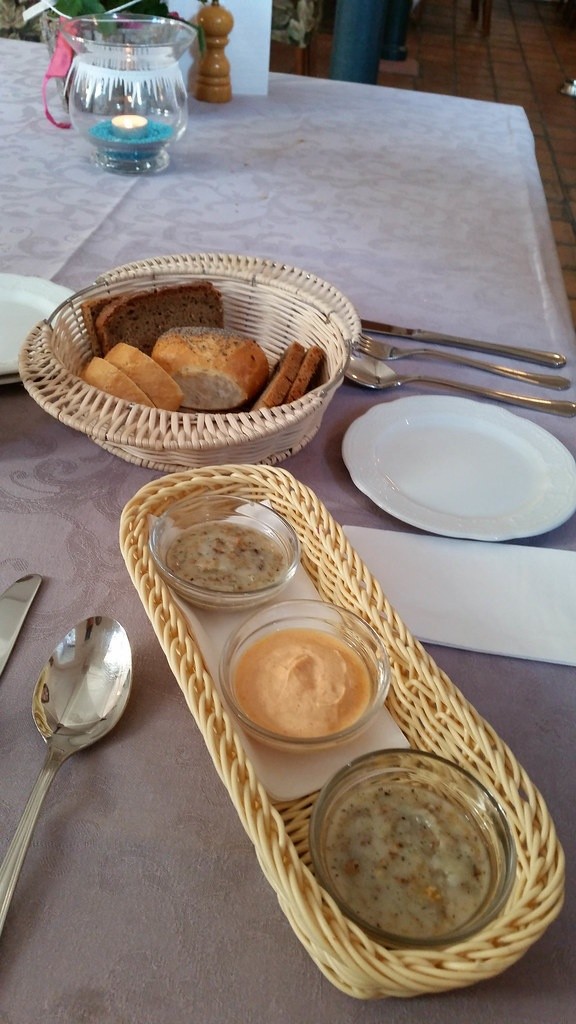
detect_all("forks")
[356,334,571,391]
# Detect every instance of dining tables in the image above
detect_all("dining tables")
[0,38,576,1023]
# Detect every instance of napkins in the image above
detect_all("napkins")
[341,525,576,668]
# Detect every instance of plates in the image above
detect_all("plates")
[342,393,576,541]
[1,273,88,385]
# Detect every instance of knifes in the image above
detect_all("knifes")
[0,574,41,674]
[360,319,567,367]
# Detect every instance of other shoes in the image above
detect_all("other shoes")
[378,38,407,60]
[560,79,576,98]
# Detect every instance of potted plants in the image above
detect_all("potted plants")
[40,0,169,114]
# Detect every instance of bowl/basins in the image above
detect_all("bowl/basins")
[219,598,390,752]
[308,747,515,947]
[152,495,301,611]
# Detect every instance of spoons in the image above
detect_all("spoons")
[0,616,132,932]
[344,351,576,418]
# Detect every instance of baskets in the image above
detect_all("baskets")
[18,252,362,472]
[118,463,566,1000]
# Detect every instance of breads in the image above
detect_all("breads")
[102,343,185,412]
[81,356,157,408]
[246,341,322,411]
[150,324,270,411]
[80,279,224,357]
